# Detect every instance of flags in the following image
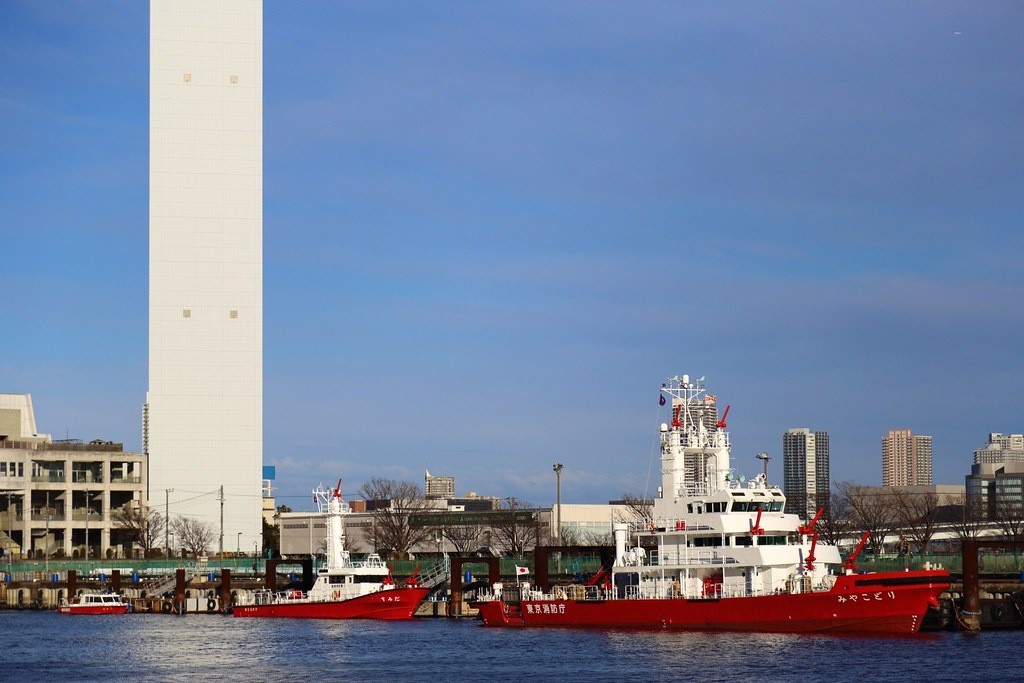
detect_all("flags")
[518,567,529,574]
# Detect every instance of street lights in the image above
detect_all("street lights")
[237,533,242,557]
[552,462,564,573]
[755,453,771,487]
[519,539,524,561]
[170,532,174,553]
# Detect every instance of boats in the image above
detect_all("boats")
[464,369,951,635]
[56,592,128,614]
[231,477,432,620]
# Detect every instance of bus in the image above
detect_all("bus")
[865,548,885,554]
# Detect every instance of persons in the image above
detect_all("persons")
[553,584,589,600]
[674,580,680,598]
